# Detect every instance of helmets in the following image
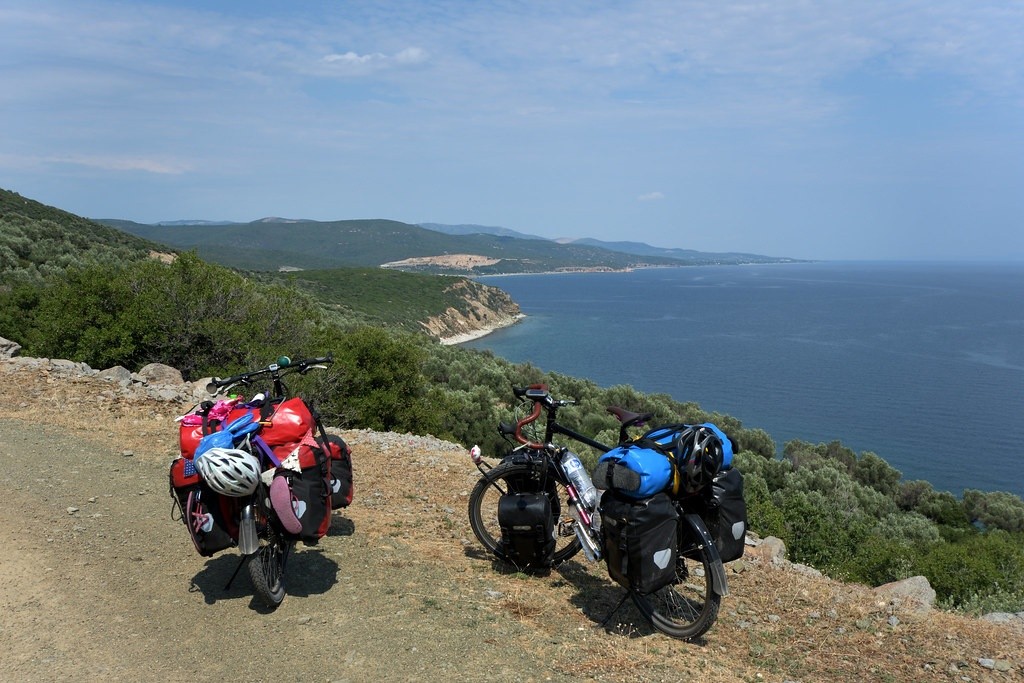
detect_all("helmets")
[195,448,262,498]
[676,425,723,494]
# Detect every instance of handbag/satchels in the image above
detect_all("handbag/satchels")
[500,450,561,525]
[171,457,240,557]
[599,422,733,499]
[678,468,748,564]
[501,494,556,570]
[600,490,679,596]
[179,397,316,458]
[316,434,354,510]
[267,438,332,545]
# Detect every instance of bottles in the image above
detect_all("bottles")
[592,489,605,531]
[560,446,595,511]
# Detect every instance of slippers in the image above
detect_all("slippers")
[269,476,303,534]
[188,491,210,553]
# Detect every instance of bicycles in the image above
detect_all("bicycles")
[169,353,357,610]
[468,388,747,641]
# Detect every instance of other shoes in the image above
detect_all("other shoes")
[592,461,640,491]
[727,435,738,454]
